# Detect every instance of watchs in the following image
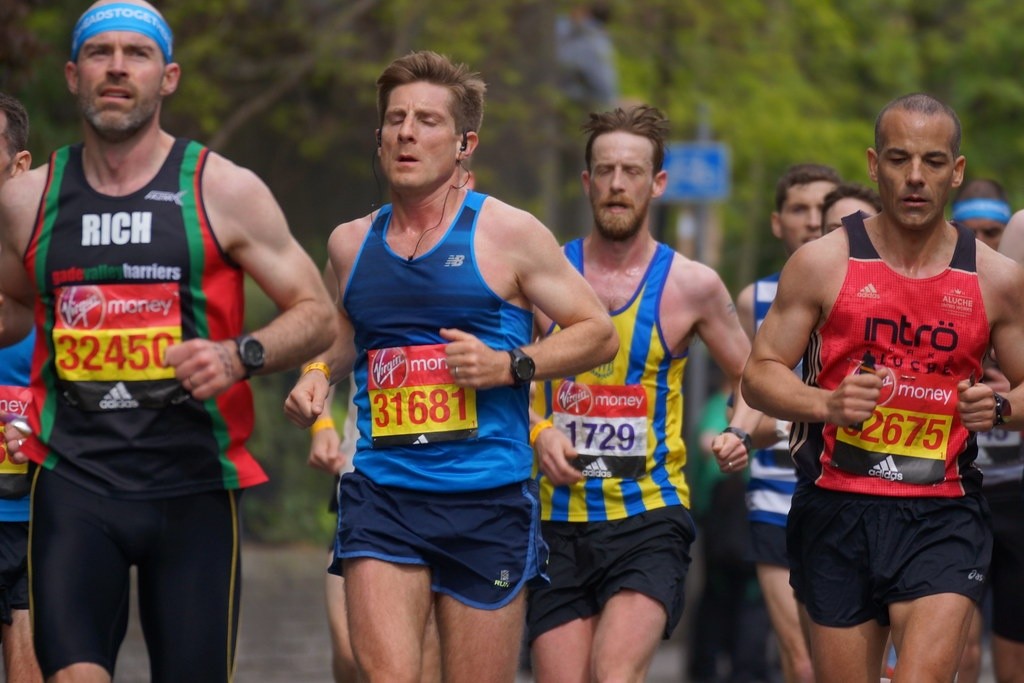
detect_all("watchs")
[508,348,535,390]
[234,333,266,381]
[992,391,1012,429]
[723,427,752,454]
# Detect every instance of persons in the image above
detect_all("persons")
[307,154,475,683]
[0,93,44,683]
[283,50,620,683]
[725,92,1024,683]
[0,0,339,683]
[526,103,764,683]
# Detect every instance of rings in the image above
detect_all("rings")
[18,439,22,446]
[455,366,458,378]
[729,463,733,470]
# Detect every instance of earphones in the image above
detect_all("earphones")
[377,129,382,147]
[460,128,468,151]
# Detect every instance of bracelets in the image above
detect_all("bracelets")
[530,420,553,448]
[310,418,334,437]
[304,362,330,381]
